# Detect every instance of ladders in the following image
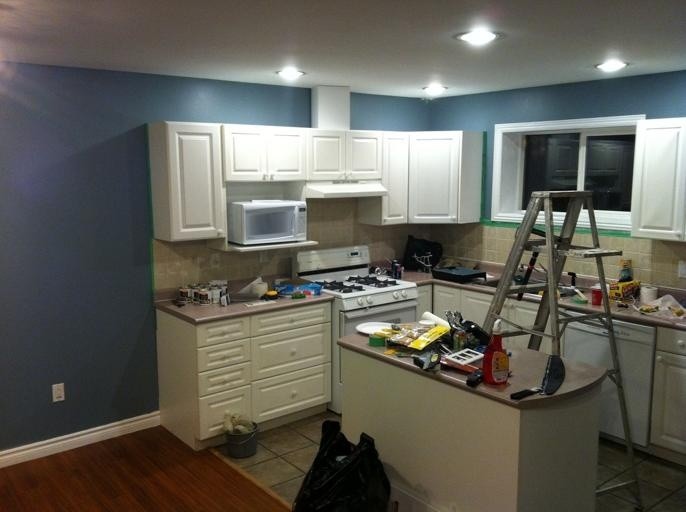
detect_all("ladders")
[483,190,643,512]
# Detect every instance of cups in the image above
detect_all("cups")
[592,289,603,305]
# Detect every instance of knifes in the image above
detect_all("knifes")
[511,385,544,401]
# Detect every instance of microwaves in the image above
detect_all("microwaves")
[227,198,308,245]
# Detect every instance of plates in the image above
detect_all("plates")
[356,321,392,335]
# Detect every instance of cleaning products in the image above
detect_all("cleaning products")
[482,319,509,387]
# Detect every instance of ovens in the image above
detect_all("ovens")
[332,287,421,415]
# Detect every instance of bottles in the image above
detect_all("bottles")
[392,259,401,279]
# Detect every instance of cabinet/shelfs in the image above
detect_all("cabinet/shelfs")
[358,132,410,225]
[415,284,433,321]
[461,287,566,356]
[631,117,686,241]
[147,119,228,241]
[221,124,306,183]
[649,323,686,467]
[433,283,461,326]
[408,131,484,226]
[151,274,331,451]
[307,128,384,180]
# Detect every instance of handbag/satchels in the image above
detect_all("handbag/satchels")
[293,420,390,512]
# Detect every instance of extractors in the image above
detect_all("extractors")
[306,180,389,199]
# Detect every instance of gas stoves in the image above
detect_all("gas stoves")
[314,274,418,299]
